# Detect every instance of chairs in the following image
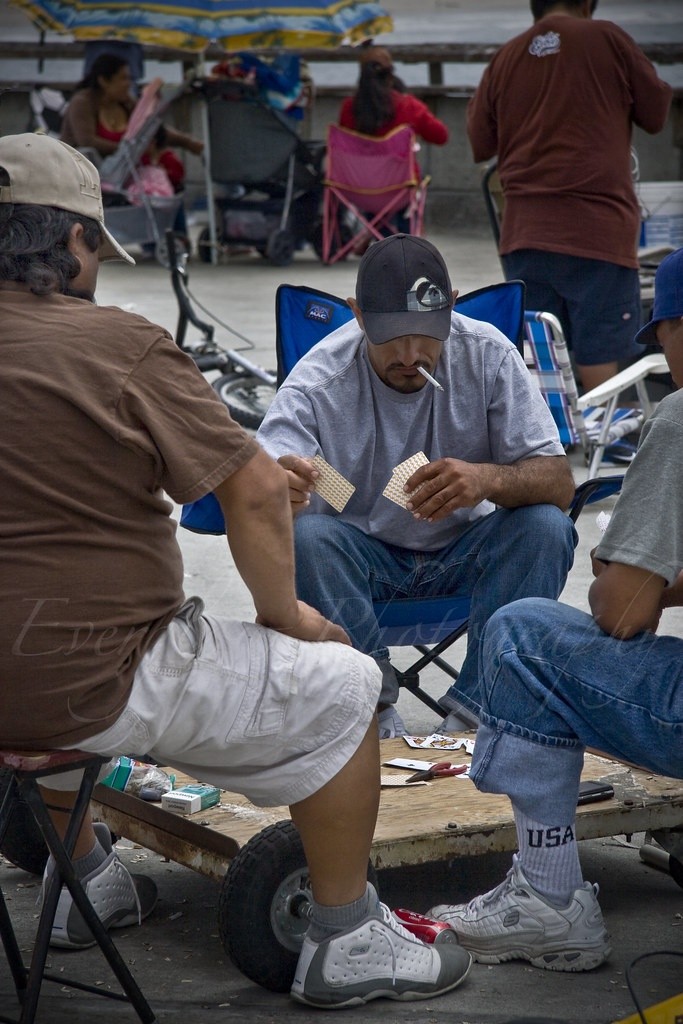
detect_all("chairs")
[177,280,626,721]
[519,310,671,482]
[321,124,431,267]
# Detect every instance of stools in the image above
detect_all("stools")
[0,746,158,1024]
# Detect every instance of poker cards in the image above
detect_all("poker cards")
[449,762,471,780]
[309,455,356,512]
[380,774,427,786]
[384,758,437,771]
[400,733,475,755]
[382,451,431,513]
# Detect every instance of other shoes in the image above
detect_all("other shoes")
[429,713,472,734]
[376,706,412,738]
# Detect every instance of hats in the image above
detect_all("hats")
[634,247,683,346]
[0,132,135,265]
[359,46,392,71]
[356,232,453,345]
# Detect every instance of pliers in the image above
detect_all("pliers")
[404,760,467,782]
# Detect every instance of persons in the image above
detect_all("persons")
[0,128,474,1008]
[423,245,683,973]
[465,0,683,471]
[256,234,579,743]
[58,50,203,257]
[337,45,449,239]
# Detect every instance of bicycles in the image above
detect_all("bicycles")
[161,226,277,432]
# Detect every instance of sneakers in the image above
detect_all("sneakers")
[32,821,158,948]
[425,853,614,972]
[290,882,473,1006]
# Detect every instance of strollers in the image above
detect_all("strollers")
[181,69,327,267]
[75,77,192,271]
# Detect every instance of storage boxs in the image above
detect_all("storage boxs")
[161,784,220,814]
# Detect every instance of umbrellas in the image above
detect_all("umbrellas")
[14,0,393,267]
